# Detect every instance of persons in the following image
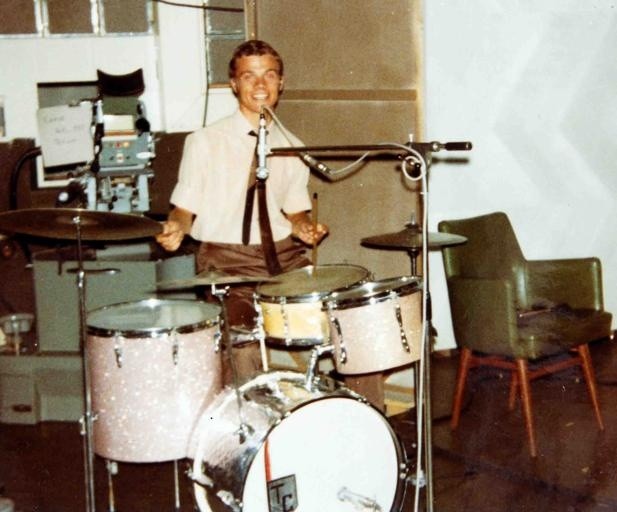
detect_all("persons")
[155,40,329,390]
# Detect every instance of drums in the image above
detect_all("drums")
[253,262,373,353]
[185,346,408,512]
[320,274,433,376]
[81,297,222,463]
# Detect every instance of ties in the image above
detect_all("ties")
[241,130,282,277]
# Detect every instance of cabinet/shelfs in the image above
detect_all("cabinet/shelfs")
[1,238,202,428]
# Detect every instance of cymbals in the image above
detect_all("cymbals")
[359,209,468,252]
[0,206,165,242]
[144,270,281,293]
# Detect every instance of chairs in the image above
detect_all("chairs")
[439,211,612,461]
